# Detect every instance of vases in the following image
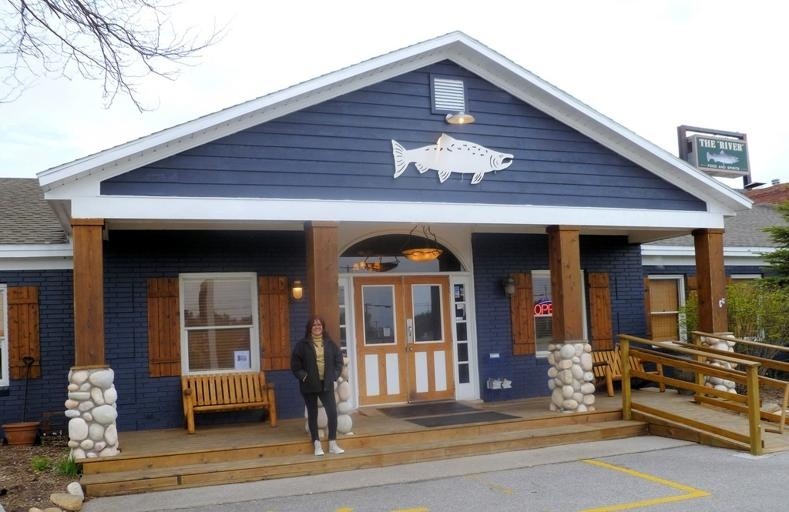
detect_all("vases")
[0,419,41,446]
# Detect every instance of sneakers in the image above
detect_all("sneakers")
[328,439,345,455]
[313,440,325,456]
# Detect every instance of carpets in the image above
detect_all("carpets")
[373,401,482,420]
[404,409,522,429]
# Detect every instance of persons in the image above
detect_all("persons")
[290,314,345,457]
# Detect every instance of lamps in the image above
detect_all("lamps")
[290,278,304,301]
[445,111,475,126]
[352,259,381,273]
[365,243,400,272]
[502,277,517,296]
[400,224,445,263]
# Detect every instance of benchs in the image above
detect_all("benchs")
[180,370,278,435]
[589,346,667,397]
